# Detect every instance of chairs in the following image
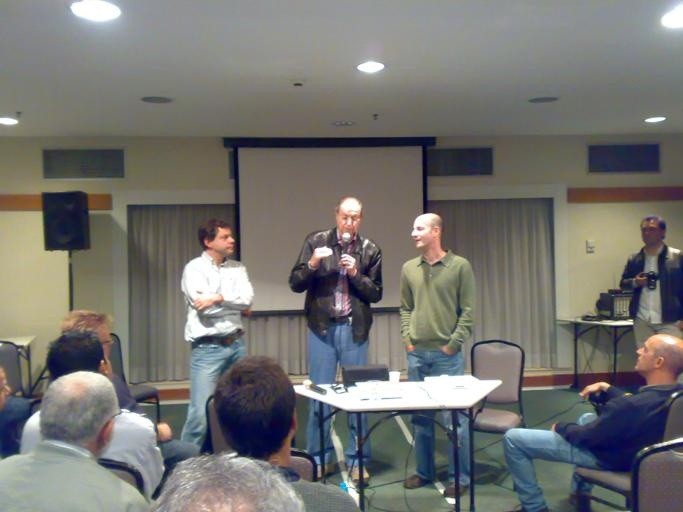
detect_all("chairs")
[110,333,161,426]
[472,340,523,432]
[577,389,682,510]
[0,340,40,410]
[633,439,683,512]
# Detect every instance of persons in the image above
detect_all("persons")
[399,212,476,497]
[619,216,682,384]
[213,355,359,512]
[0,372,151,512]
[0,367,34,461]
[60,309,202,487]
[503,335,682,512]
[181,216,255,456]
[152,454,305,512]
[20,331,165,503]
[288,196,382,484]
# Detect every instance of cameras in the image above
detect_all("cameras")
[641,271,661,290]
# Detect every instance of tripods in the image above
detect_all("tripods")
[28,254,73,397]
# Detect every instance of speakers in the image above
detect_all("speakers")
[42,191,91,250]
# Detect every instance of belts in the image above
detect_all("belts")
[328,313,352,324]
[197,330,244,346]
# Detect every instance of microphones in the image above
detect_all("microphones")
[342,233,351,267]
[302,379,327,394]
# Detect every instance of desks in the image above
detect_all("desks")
[293,374,501,511]
[557,314,634,388]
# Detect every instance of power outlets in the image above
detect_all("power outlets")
[585,238,597,256]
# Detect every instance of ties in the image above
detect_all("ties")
[336,251,352,317]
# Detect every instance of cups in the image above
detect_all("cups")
[389,371,400,385]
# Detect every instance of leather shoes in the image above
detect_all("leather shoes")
[443,482,466,498]
[346,464,370,482]
[317,465,335,478]
[403,475,427,489]
